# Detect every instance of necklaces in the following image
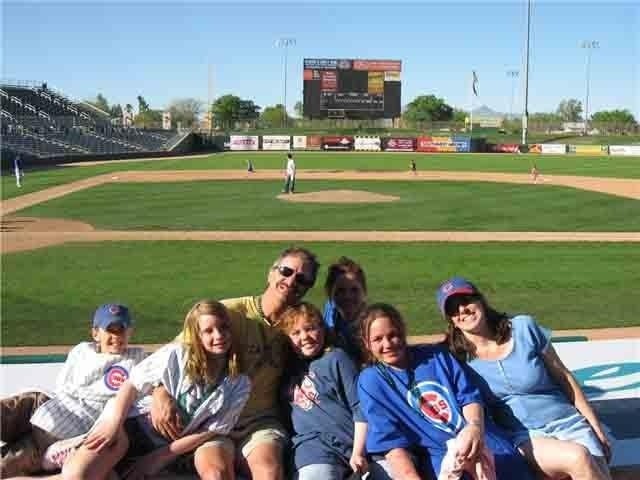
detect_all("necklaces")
[256,289,271,327]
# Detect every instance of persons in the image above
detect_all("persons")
[321,254,370,365]
[0,302,156,478]
[243,157,261,177]
[280,147,299,195]
[276,302,391,480]
[529,162,544,186]
[11,154,27,188]
[434,275,618,479]
[148,245,321,479]
[40,298,255,480]
[407,156,420,175]
[354,301,538,479]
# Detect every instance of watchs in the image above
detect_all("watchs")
[466,418,483,426]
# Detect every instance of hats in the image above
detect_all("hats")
[92,303,131,330]
[436,277,481,319]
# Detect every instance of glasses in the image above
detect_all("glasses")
[273,265,311,288]
